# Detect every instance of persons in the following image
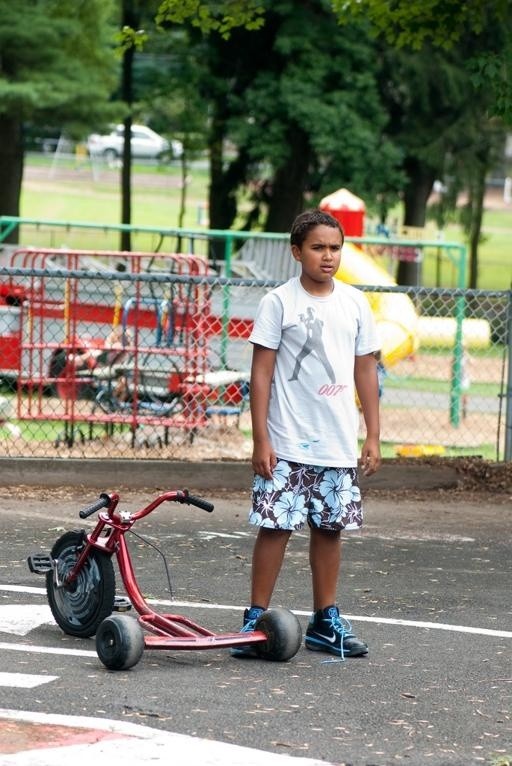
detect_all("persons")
[229,208,382,658]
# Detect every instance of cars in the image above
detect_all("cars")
[86,123,183,164]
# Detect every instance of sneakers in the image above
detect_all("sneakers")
[230,606,266,656]
[305,606,368,656]
